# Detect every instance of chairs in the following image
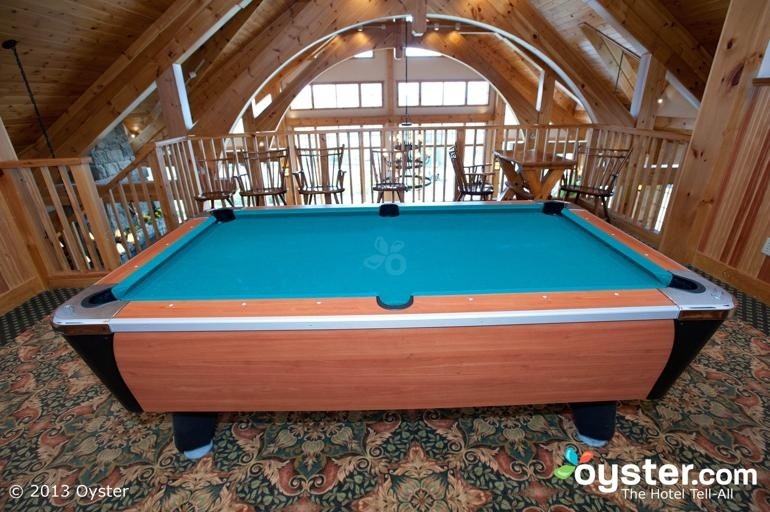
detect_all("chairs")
[193,144,409,213]
[561,145,633,224]
[448,146,496,201]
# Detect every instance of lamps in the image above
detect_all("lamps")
[380,17,432,193]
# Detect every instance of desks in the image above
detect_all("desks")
[492,148,577,201]
[53,199,739,461]
